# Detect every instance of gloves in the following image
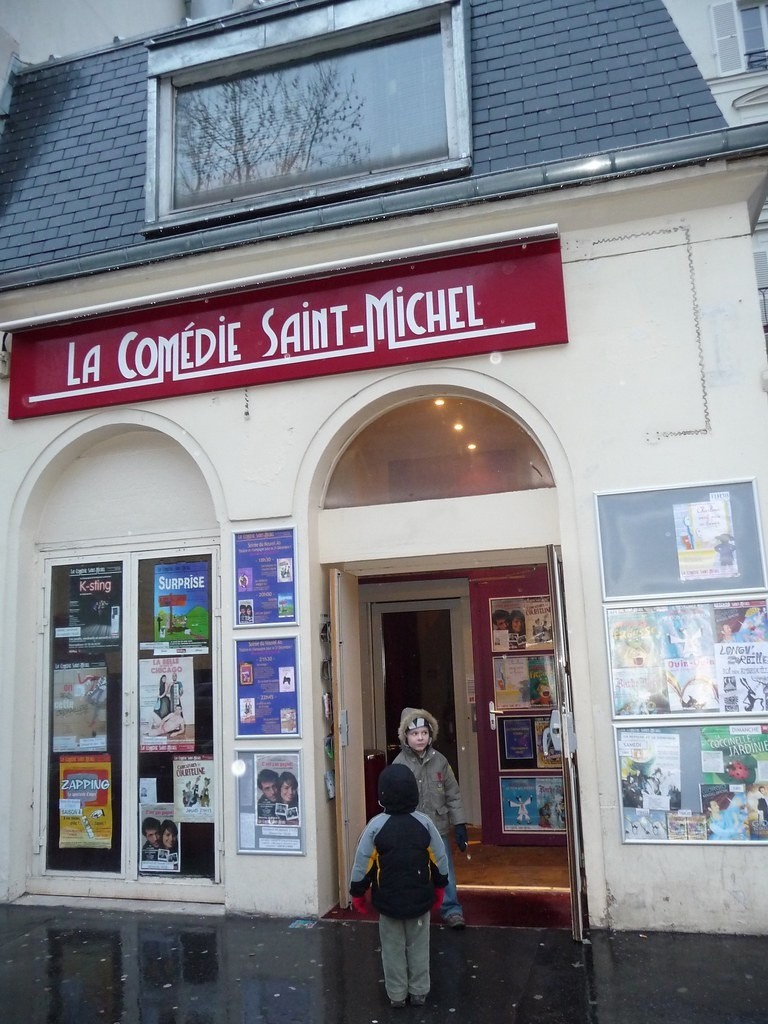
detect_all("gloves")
[455,824,468,852]
[351,896,369,913]
[432,887,444,910]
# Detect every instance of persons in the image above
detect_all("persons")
[349,764,449,1009]
[391,708,468,931]
[142,817,178,860]
[706,799,743,840]
[257,769,298,817]
[720,623,737,643]
[492,610,525,635]
[757,786,768,825]
[240,605,252,621]
[145,672,185,737]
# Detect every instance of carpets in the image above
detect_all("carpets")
[321,889,573,927]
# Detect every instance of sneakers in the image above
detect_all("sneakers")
[410,993,428,1006]
[390,999,408,1007]
[448,915,465,929]
